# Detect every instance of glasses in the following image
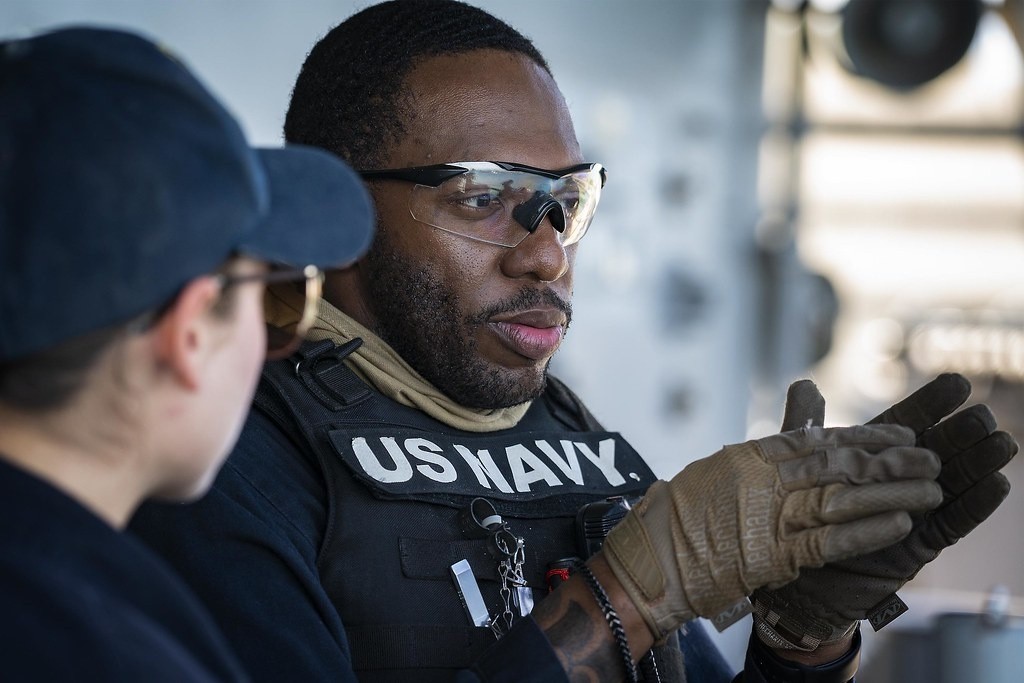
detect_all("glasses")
[361,161,606,248]
[142,266,321,360]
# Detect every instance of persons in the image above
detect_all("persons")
[0,24,380,683]
[130,2,1021,683]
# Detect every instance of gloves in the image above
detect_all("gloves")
[603,424,943,637]
[750,372,1019,651]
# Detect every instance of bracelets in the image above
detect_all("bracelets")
[554,556,663,683]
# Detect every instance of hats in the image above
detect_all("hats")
[0,25,373,352]
